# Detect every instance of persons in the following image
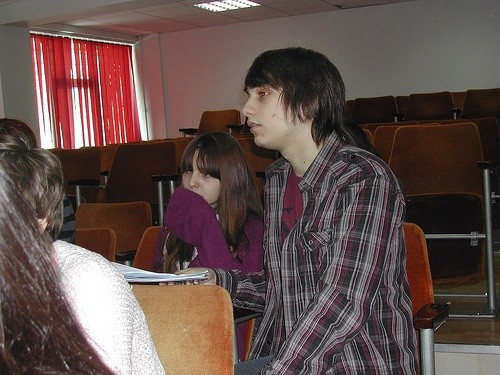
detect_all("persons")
[0,118,166,375]
[151,131,265,363]
[234,47,418,375]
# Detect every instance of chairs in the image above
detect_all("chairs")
[48,87,499,374]
